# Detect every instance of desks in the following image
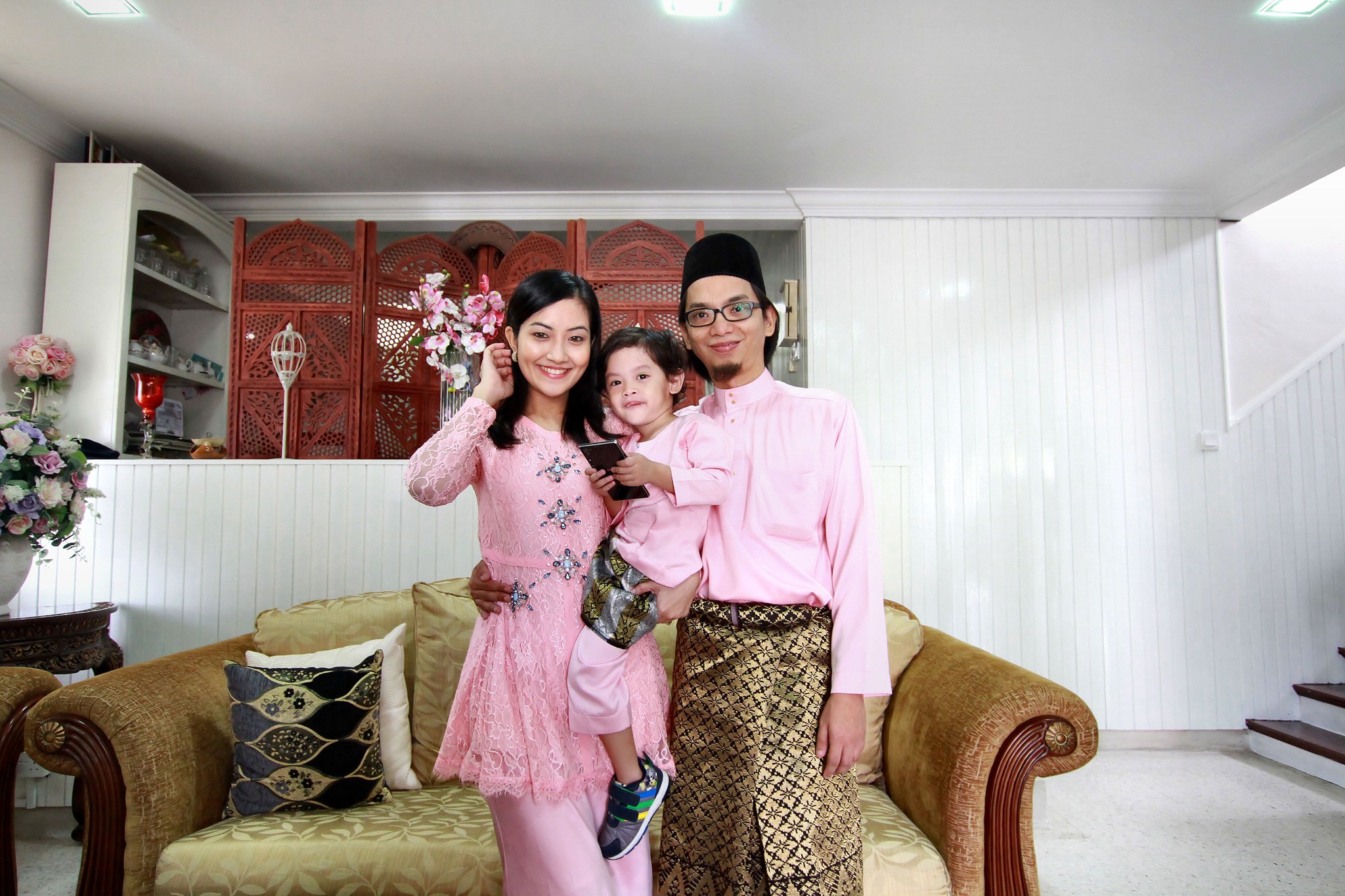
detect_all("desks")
[0,601,124,841]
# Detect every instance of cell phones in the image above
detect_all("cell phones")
[579,440,650,501]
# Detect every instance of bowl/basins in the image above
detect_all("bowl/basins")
[129,339,142,351]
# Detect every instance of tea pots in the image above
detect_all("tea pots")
[141,343,165,363]
[178,356,193,372]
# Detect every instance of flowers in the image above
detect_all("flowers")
[0,333,107,565]
[409,269,505,393]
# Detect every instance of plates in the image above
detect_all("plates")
[129,350,150,359]
[130,308,172,351]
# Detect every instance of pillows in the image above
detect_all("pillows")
[855,606,923,783]
[245,622,422,790]
[220,649,393,820]
[410,577,480,788]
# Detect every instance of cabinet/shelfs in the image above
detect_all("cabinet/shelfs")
[37,163,234,459]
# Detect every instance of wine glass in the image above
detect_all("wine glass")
[131,373,168,459]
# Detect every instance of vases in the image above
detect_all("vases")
[440,351,480,431]
[0,533,33,617]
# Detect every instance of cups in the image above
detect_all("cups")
[190,362,204,373]
[134,236,210,294]
[162,345,180,368]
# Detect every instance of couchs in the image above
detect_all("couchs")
[23,577,1098,896]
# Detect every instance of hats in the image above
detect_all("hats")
[681,233,766,292]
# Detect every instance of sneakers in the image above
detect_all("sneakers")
[597,751,670,860]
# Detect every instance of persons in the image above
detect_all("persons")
[405,272,735,896]
[469,229,894,896]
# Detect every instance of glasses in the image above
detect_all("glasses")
[685,301,763,327]
